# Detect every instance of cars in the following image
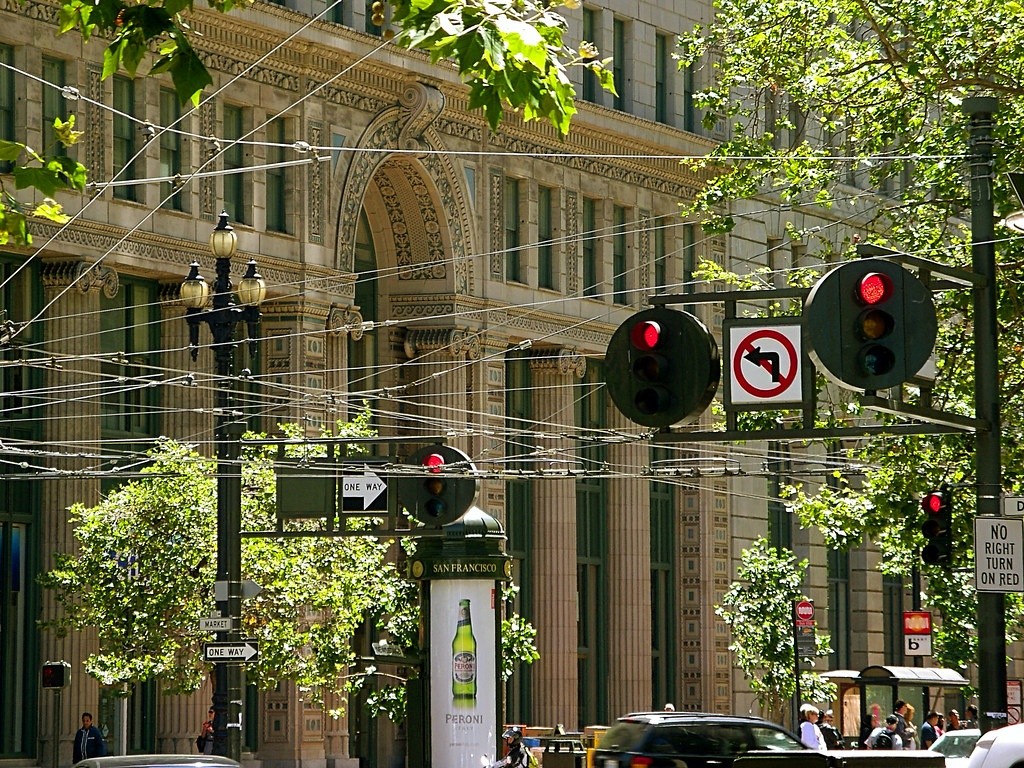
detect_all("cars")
[927,728,981,768]
[68,753,243,767]
[968,722,1024,768]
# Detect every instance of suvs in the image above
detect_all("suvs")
[590,711,816,767]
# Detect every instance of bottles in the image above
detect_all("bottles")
[452,600,478,698]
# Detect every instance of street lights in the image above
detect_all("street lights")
[177,207,267,767]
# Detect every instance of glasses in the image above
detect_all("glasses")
[209,711,215,714]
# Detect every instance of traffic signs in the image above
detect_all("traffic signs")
[201,641,259,663]
[337,457,399,520]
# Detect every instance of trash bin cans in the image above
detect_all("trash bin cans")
[542,740,588,768]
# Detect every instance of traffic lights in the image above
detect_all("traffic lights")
[41,658,72,690]
[398,442,481,526]
[920,490,955,566]
[801,257,939,392]
[602,306,721,430]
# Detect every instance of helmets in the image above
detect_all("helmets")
[505,727,523,746]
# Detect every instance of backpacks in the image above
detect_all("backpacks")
[873,728,896,749]
[521,746,539,768]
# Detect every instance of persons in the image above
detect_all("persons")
[73,712,104,763]
[946,709,963,732]
[664,703,676,711]
[197,705,217,755]
[963,704,979,729]
[864,714,903,750]
[920,711,944,750]
[904,703,921,750]
[490,728,529,768]
[798,703,846,750]
[892,700,916,750]
